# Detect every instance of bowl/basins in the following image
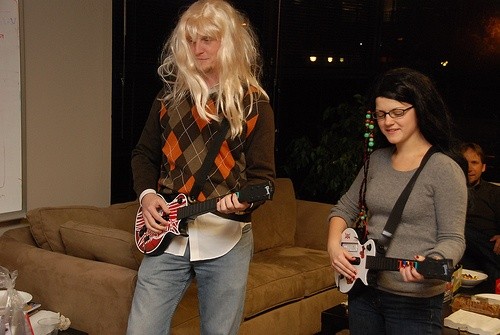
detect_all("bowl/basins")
[451,268,488,288]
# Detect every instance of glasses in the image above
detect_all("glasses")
[371,104,415,119]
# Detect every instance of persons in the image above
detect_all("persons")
[458,142,500,293]
[125,0,274,335]
[327,67,470,335]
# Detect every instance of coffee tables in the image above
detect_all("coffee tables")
[321,287,500,335]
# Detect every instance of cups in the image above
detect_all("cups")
[37,318,61,335]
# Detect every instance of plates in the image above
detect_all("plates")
[471,294,500,305]
[5,317,55,335]
[0,290,33,309]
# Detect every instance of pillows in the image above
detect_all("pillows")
[25,206,141,271]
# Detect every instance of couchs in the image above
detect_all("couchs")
[0,178,348,335]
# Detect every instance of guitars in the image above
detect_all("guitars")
[333,226,455,297]
[134,180,275,257]
